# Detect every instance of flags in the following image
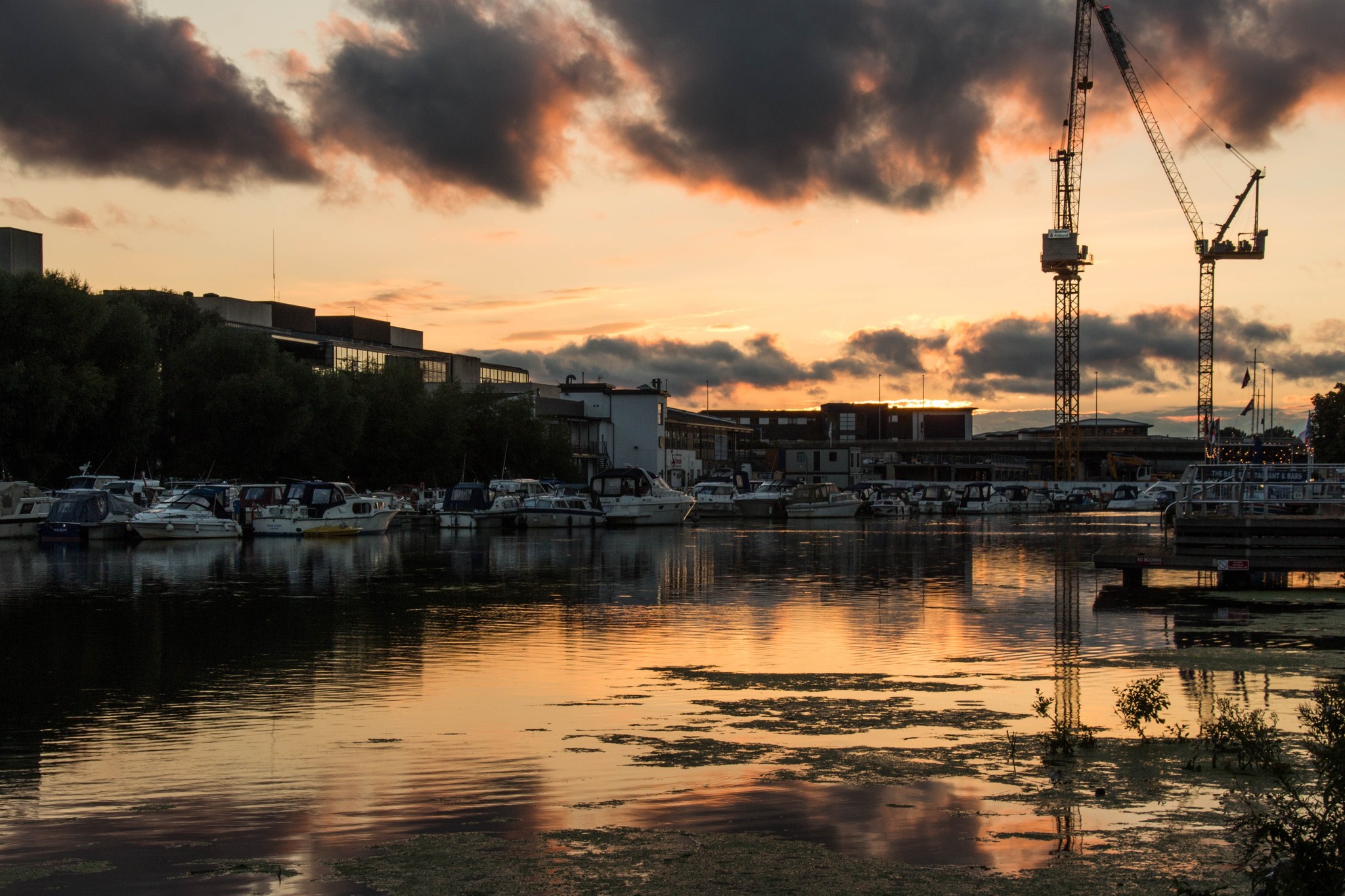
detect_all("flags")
[1241,398,1253,416]
[1242,369,1250,388]
[1202,413,1219,444]
[1299,414,1312,451]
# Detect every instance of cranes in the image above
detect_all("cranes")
[1040,1,1098,482]
[1088,0,1272,451]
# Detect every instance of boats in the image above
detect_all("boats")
[683,474,1177,513]
[0,464,696,545]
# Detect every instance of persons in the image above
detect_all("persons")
[1054,484,1060,490]
